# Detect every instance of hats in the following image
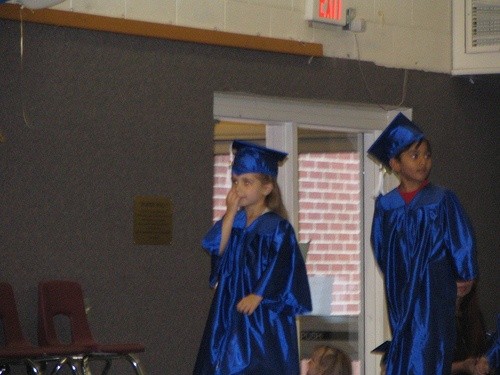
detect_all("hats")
[368,112,425,171]
[232,140,287,176]
[370,341,391,365]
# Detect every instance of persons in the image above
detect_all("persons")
[366,112,478,375]
[450,280,500,375]
[193,139,313,375]
[307,344,352,375]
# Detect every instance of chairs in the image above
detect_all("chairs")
[38,282,145,375]
[0,283,91,375]
